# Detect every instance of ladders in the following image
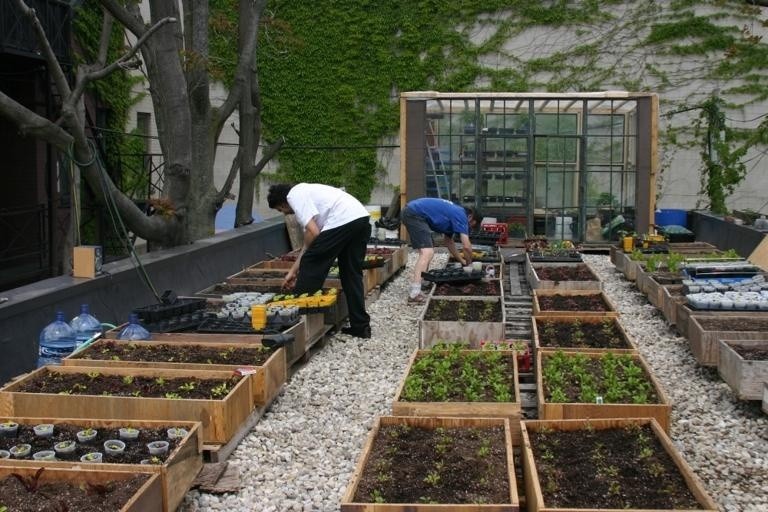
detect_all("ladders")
[425,120,451,202]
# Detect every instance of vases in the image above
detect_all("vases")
[461,126,526,204]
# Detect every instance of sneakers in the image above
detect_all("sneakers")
[408,294,426,305]
[341,326,371,338]
[422,282,434,290]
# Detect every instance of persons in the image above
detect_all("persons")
[400,197,482,305]
[267,183,371,338]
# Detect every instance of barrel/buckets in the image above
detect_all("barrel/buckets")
[116,313,152,343]
[68,304,104,349]
[554,216,573,241]
[35,311,77,369]
[364,205,382,238]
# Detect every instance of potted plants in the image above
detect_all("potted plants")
[594,191,617,228]
[514,111,529,135]
[458,108,485,135]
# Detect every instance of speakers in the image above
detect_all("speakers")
[73,246,104,278]
